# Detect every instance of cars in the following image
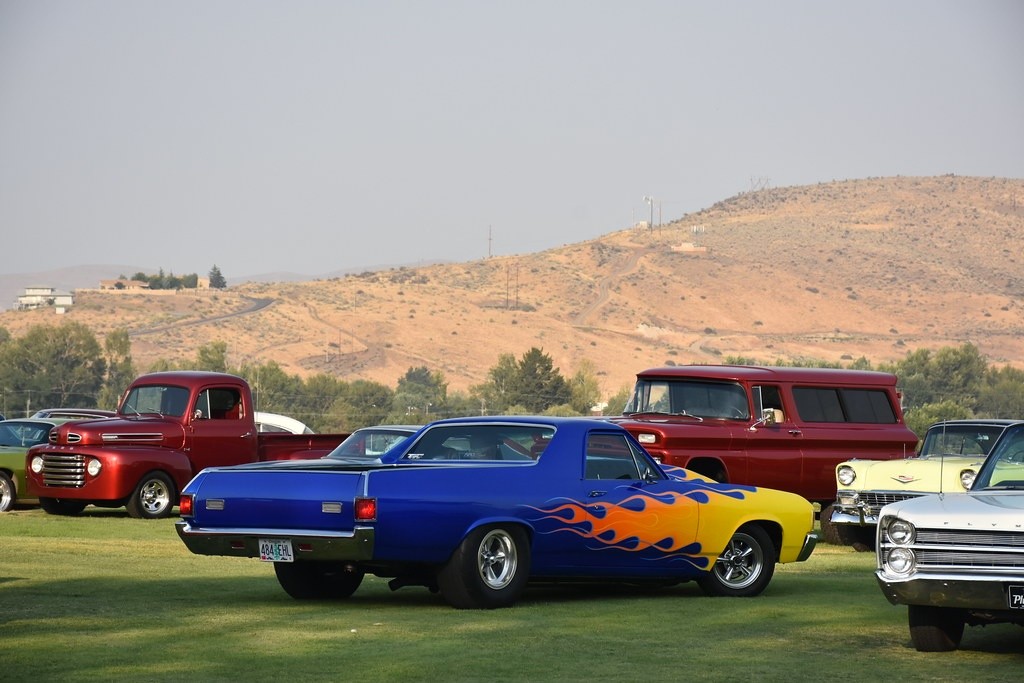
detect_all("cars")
[173,415,821,614]
[828,417,1024,555]
[225,424,534,469]
[253,411,315,437]
[874,422,1024,651]
[0,417,77,510]
[16,406,119,443]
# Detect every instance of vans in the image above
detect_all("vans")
[530,364,924,525]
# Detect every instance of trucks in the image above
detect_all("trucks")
[23,370,367,520]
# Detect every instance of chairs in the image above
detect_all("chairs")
[468,437,498,460]
